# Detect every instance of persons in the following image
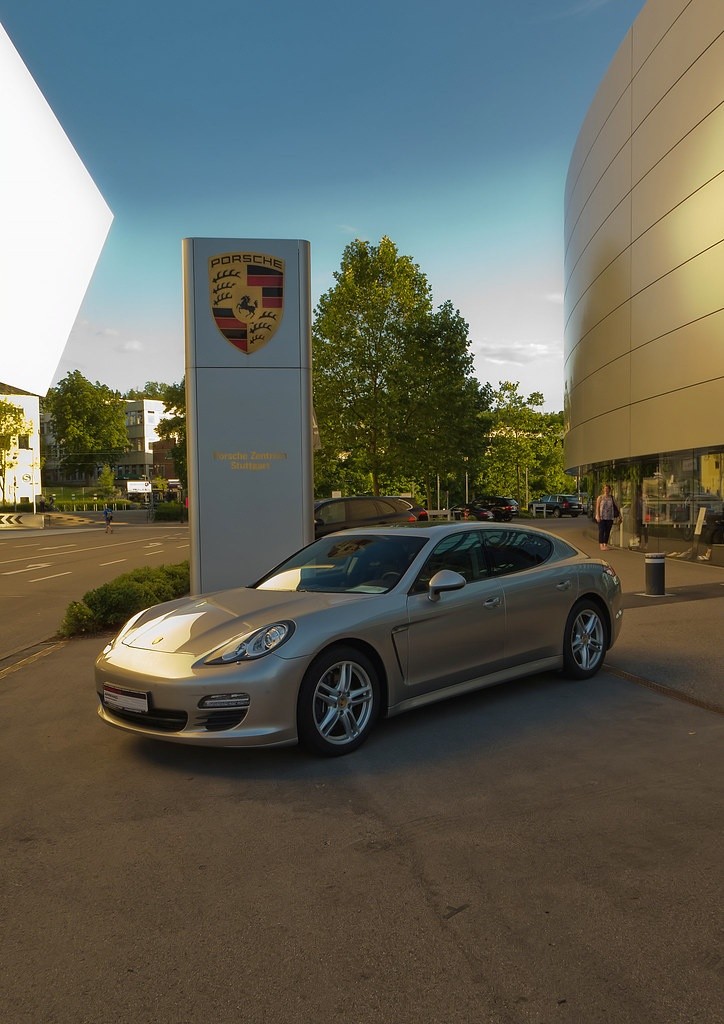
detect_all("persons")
[103,505,114,534]
[40,494,54,513]
[596,485,614,550]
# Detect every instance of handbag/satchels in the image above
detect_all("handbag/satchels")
[611,495,619,518]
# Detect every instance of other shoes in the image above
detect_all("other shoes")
[599,543,608,550]
[111,530,113,534]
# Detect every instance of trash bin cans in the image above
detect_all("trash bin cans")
[644,552,666,595]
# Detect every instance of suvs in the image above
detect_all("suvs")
[472,496,519,521]
[528,494,584,518]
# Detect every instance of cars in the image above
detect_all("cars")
[621,494,723,521]
[398,498,429,522]
[94,520,624,760]
[444,503,494,520]
[314,496,418,540]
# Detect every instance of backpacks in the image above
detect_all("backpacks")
[106,509,112,521]
